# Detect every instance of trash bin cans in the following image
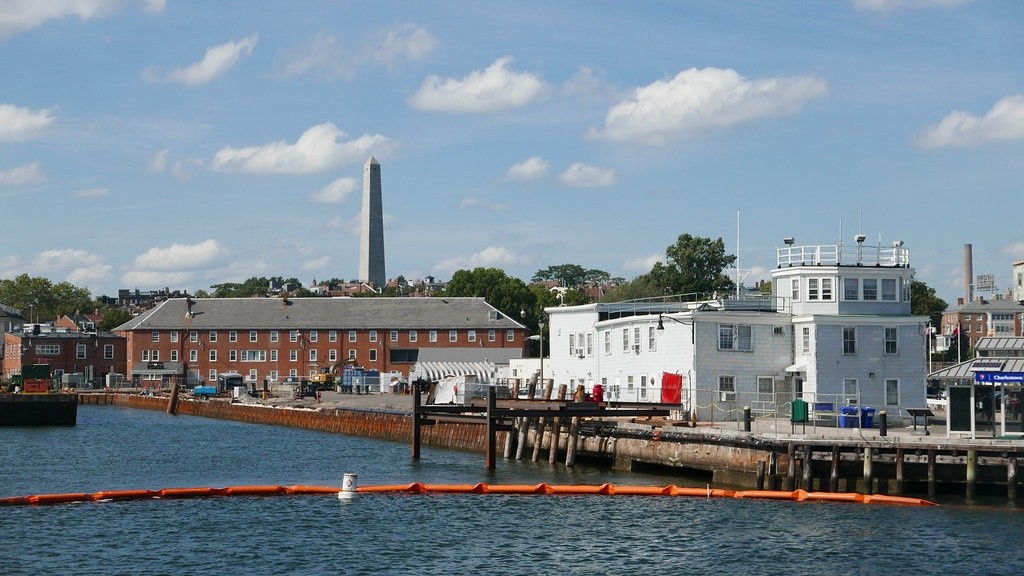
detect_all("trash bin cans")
[839,407,876,428]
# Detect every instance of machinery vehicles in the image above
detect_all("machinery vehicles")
[308,356,358,390]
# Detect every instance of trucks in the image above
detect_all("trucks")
[194,371,243,400]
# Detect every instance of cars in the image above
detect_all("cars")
[926,386,1003,412]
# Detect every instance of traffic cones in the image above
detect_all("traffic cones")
[316,394,321,403]
[692,408,697,422]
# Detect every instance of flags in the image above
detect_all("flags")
[660,372,683,404]
[926,327,929,335]
[953,326,958,336]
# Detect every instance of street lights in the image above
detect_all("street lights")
[537,314,545,402]
[556,293,563,307]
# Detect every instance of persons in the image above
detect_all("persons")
[1004,393,1011,420]
[1010,393,1020,420]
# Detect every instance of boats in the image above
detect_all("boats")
[1,361,77,427]
